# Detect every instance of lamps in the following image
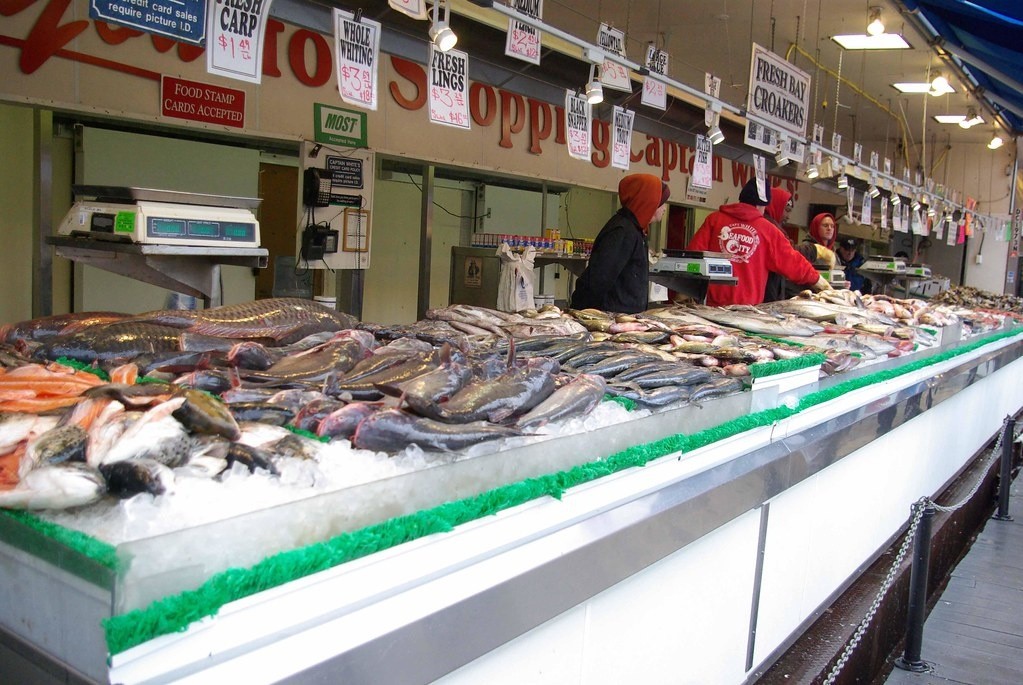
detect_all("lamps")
[805,153,818,178]
[428,0,458,51]
[927,200,936,216]
[890,185,900,205]
[775,141,789,166]
[910,193,921,211]
[931,68,947,89]
[946,207,954,222]
[707,112,724,145]
[586,64,604,104]
[837,166,848,188]
[868,177,880,198]
[867,6,884,35]
[958,214,966,225]
[958,105,978,129]
[991,128,1003,147]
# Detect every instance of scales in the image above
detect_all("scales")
[860,255,907,273]
[650,250,734,277]
[814,265,847,281]
[905,264,932,277]
[57,183,263,250]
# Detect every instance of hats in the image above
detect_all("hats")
[788,198,794,208]
[740,177,771,206]
[840,238,857,251]
[658,181,670,207]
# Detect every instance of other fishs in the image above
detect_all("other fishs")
[0,287,957,496]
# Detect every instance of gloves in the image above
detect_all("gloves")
[814,244,835,270]
[813,274,833,290]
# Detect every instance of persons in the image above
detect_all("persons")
[685,176,834,307]
[802,213,851,290]
[570,173,671,314]
[763,187,836,302]
[871,251,909,295]
[837,238,865,291]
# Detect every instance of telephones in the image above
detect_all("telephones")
[304,167,333,207]
